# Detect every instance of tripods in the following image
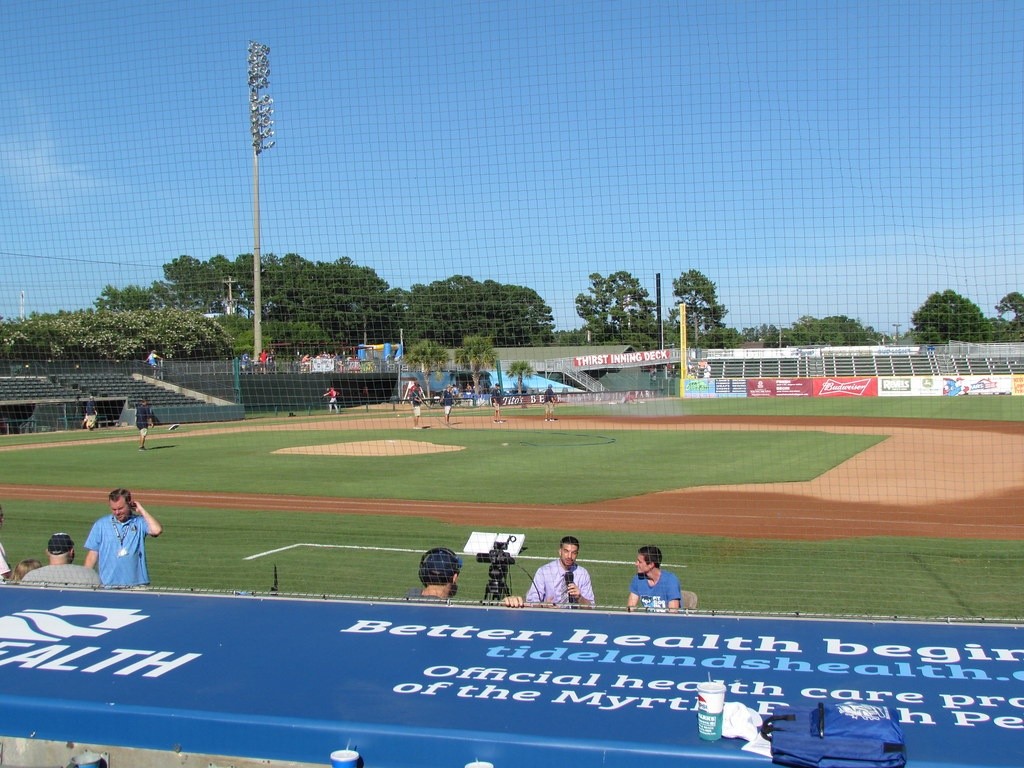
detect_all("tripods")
[482,565,510,607]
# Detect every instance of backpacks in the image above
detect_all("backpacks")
[762,703,906,768]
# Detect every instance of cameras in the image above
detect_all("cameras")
[477,542,515,565]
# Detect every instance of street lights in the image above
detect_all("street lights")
[892,323,902,345]
[247,37,275,362]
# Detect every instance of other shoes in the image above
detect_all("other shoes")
[87,426,93,431]
[493,419,502,424]
[138,446,147,451]
[545,418,554,422]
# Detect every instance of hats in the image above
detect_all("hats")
[151,350,157,353]
[446,384,453,388]
[420,548,459,583]
[548,385,552,388]
[48,532,74,554]
[495,383,499,386]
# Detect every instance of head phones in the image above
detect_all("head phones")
[418,548,463,588]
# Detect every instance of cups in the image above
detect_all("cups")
[330,750,359,768]
[464,761,493,768]
[696,683,727,741]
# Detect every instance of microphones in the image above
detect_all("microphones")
[565,571,574,604]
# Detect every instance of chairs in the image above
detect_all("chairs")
[0,373,217,409]
[682,590,697,609]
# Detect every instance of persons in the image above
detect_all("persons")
[526,536,595,610]
[135,400,154,451]
[86,396,96,431]
[302,351,340,373]
[144,350,163,377]
[83,488,163,586]
[9,559,43,582]
[0,505,12,582]
[440,384,453,424]
[627,546,683,613]
[406,548,524,608]
[22,532,103,585]
[407,381,426,429]
[544,385,556,421]
[242,349,275,374]
[491,383,504,424]
[321,386,340,413]
